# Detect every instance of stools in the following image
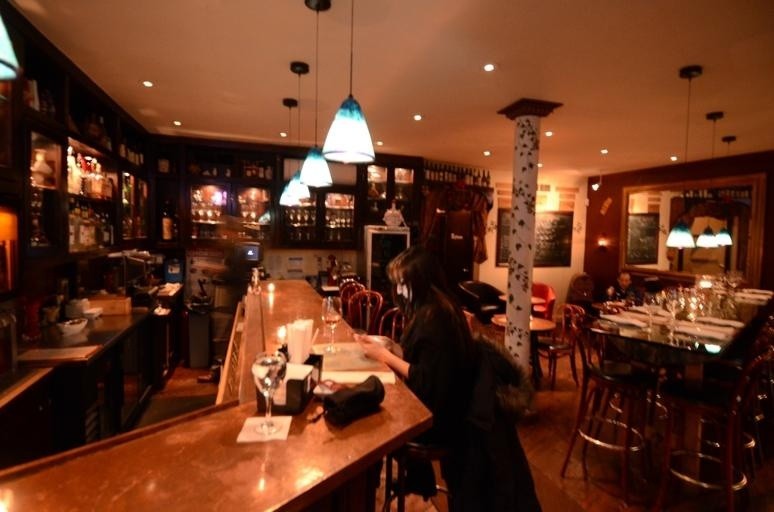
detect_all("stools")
[559,272,772,511]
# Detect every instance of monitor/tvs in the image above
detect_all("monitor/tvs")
[234,242,262,262]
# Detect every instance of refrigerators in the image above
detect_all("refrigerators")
[355,224,411,333]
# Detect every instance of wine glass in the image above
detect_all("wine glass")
[191,201,258,225]
[249,351,288,435]
[322,296,344,357]
[641,285,706,336]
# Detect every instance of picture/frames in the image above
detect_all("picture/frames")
[495,208,574,268]
[626,212,660,265]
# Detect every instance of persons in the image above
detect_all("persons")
[351,244,477,498]
[608,272,636,300]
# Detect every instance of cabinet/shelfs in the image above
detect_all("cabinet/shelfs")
[1,1,183,474]
[183,132,426,250]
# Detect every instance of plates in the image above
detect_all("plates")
[56,318,87,335]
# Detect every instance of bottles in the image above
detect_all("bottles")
[423,157,492,186]
[160,193,174,242]
[382,199,403,227]
[67,147,149,253]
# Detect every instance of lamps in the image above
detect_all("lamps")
[277,0,377,208]
[1,16,20,83]
[665,65,736,249]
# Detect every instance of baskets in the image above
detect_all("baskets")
[88,295,132,316]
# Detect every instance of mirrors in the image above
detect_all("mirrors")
[617,173,768,291]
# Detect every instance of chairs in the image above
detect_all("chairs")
[454,275,590,405]
